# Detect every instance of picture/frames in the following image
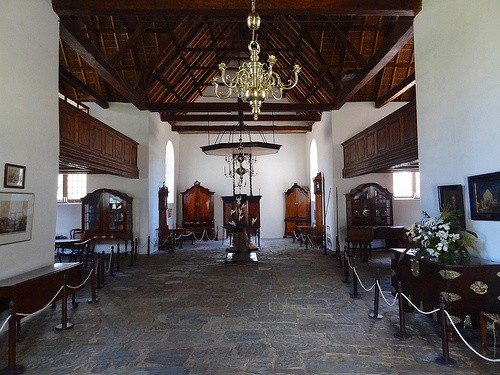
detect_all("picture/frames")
[0,192,35,246]
[436,184,466,231]
[468,171,500,222]
[3,163,26,189]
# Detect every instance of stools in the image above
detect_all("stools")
[479,309,500,359]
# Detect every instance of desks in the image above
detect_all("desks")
[297,224,314,251]
[391,247,500,340]
[169,227,186,249]
[54,238,82,262]
[0,262,85,342]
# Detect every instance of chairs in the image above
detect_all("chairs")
[69,228,97,273]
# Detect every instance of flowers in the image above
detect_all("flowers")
[400,200,479,264]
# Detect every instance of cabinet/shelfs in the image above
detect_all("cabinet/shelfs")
[220,194,261,247]
[283,182,311,238]
[159,182,170,248]
[80,188,133,257]
[343,182,394,227]
[179,180,217,241]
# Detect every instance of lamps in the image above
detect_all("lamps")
[222,113,258,193]
[211,0,302,121]
